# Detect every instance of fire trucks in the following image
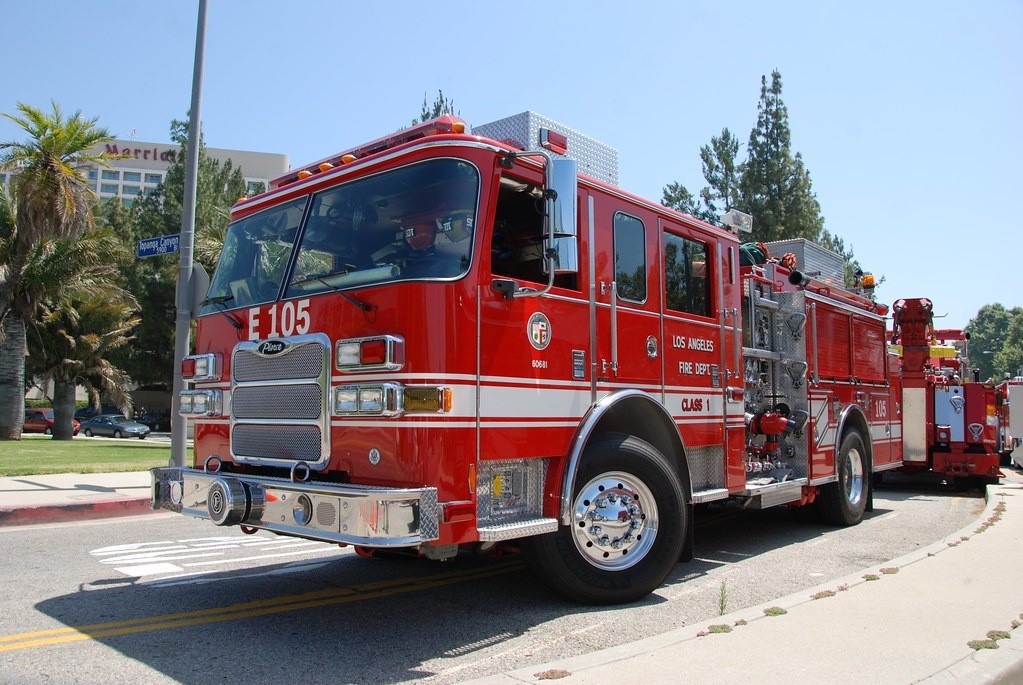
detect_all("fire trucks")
[880,296,1023,498]
[148,109,906,608]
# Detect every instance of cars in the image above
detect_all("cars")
[79,414,152,440]
[76,401,171,432]
[21,408,81,437]
